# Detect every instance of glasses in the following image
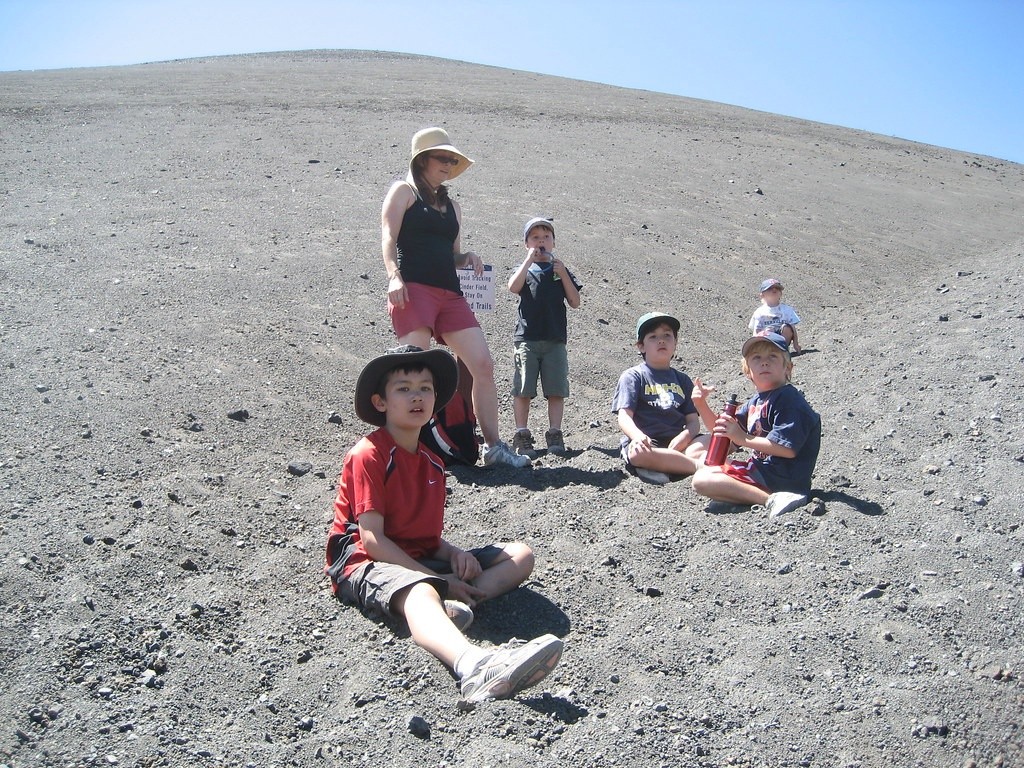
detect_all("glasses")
[421,155,458,166]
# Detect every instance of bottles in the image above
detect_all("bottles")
[703,393,738,466]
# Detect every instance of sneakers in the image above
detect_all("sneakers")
[512,429,538,459]
[441,599,472,633]
[752,490,806,519]
[545,431,565,453]
[482,443,531,467]
[461,634,564,699]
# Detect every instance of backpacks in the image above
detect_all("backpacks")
[419,383,479,465]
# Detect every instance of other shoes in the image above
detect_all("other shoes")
[637,468,669,484]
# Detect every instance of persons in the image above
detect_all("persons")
[747,278,802,355]
[611,312,715,485]
[692,330,822,519]
[382,126,530,466]
[507,217,584,461]
[323,345,562,705]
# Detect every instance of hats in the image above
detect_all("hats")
[760,279,785,293]
[635,312,680,341]
[742,330,790,355]
[406,127,474,191]
[524,217,554,243]
[355,344,459,427]
